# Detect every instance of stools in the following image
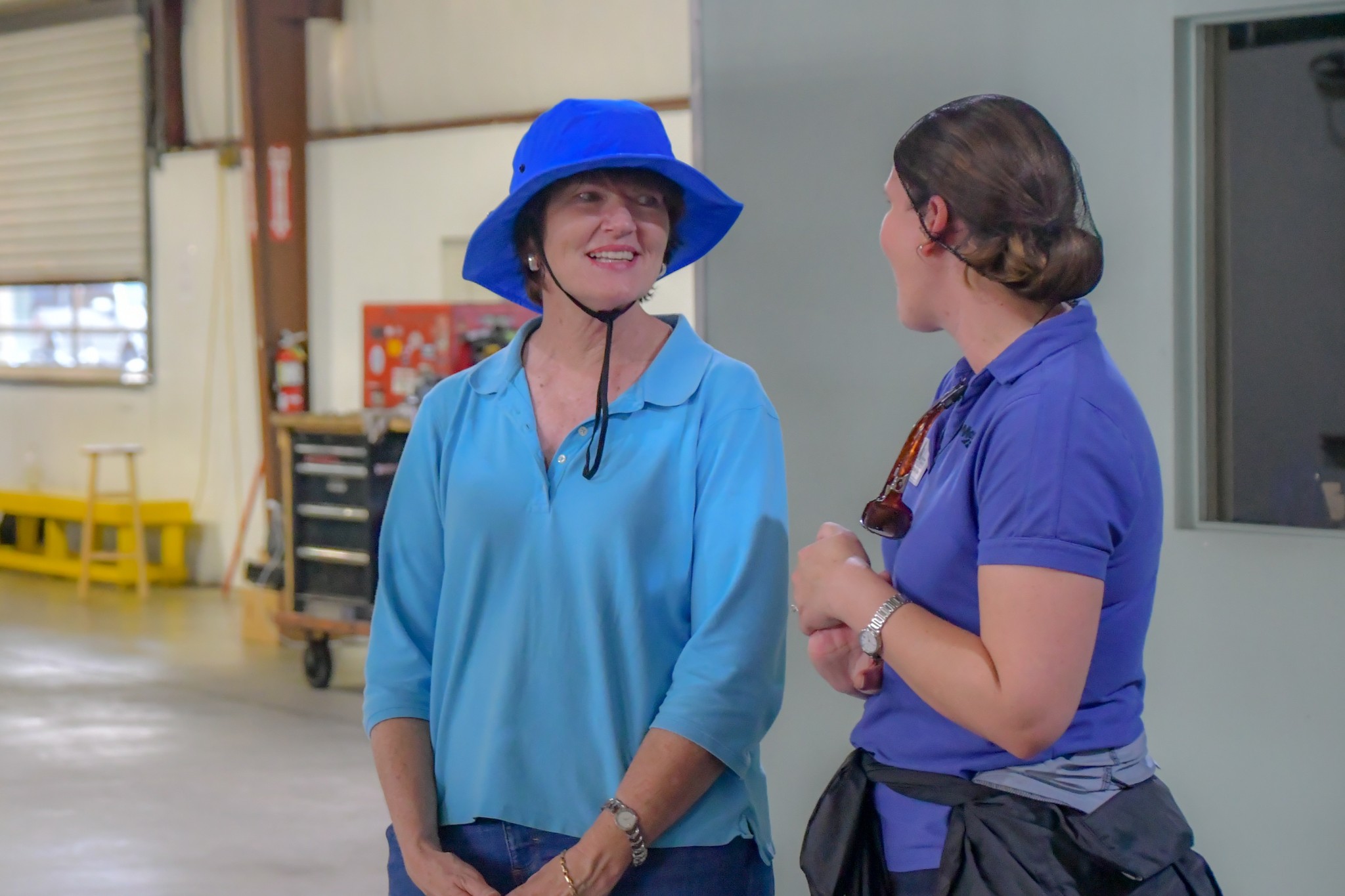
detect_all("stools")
[72,439,157,607]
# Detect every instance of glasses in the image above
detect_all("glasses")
[859,386,969,540]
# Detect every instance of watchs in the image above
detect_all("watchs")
[860,591,912,662]
[599,797,648,866]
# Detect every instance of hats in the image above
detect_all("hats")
[459,98,744,317]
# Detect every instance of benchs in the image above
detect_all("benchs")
[0,486,198,588]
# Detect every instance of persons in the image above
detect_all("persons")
[790,92,1227,896]
[364,100,788,896]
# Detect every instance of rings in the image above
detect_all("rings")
[791,604,799,613]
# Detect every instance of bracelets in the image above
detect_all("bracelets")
[560,848,582,896]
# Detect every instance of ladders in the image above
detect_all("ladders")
[78,440,151,601]
